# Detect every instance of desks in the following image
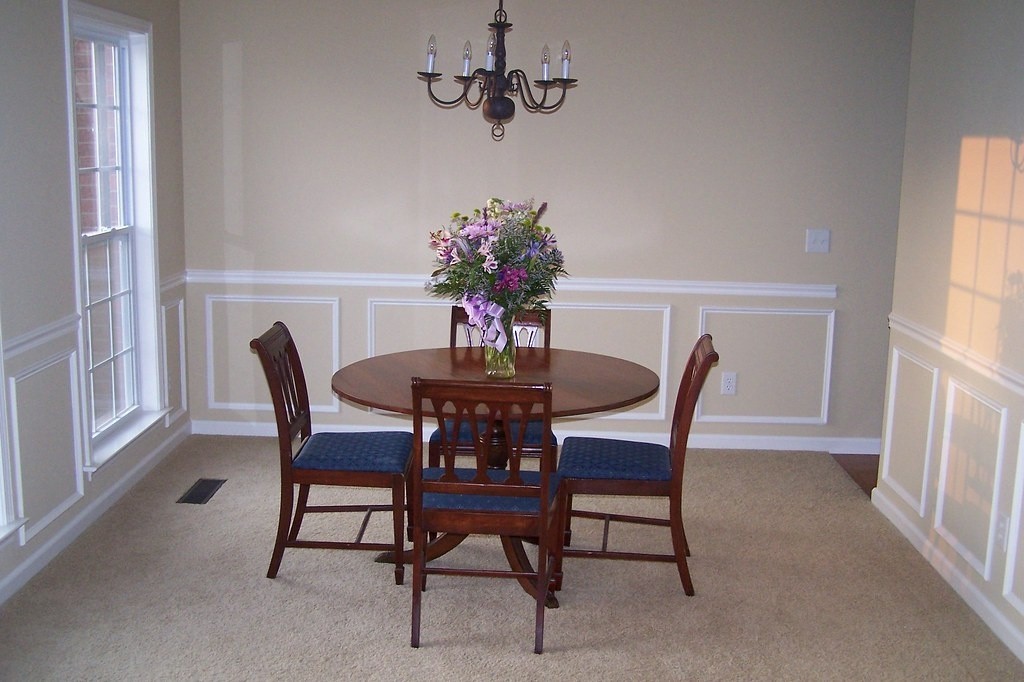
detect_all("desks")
[332,346,662,611]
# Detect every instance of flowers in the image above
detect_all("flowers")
[423,194,570,341]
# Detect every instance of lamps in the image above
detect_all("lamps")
[418,0,576,139]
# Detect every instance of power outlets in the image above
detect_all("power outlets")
[721,370,737,396]
[804,227,829,253]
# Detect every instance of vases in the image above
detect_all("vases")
[483,320,516,379]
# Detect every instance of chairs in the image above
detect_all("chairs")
[248,319,415,588]
[409,378,567,653]
[546,334,721,595]
[425,305,552,544]
